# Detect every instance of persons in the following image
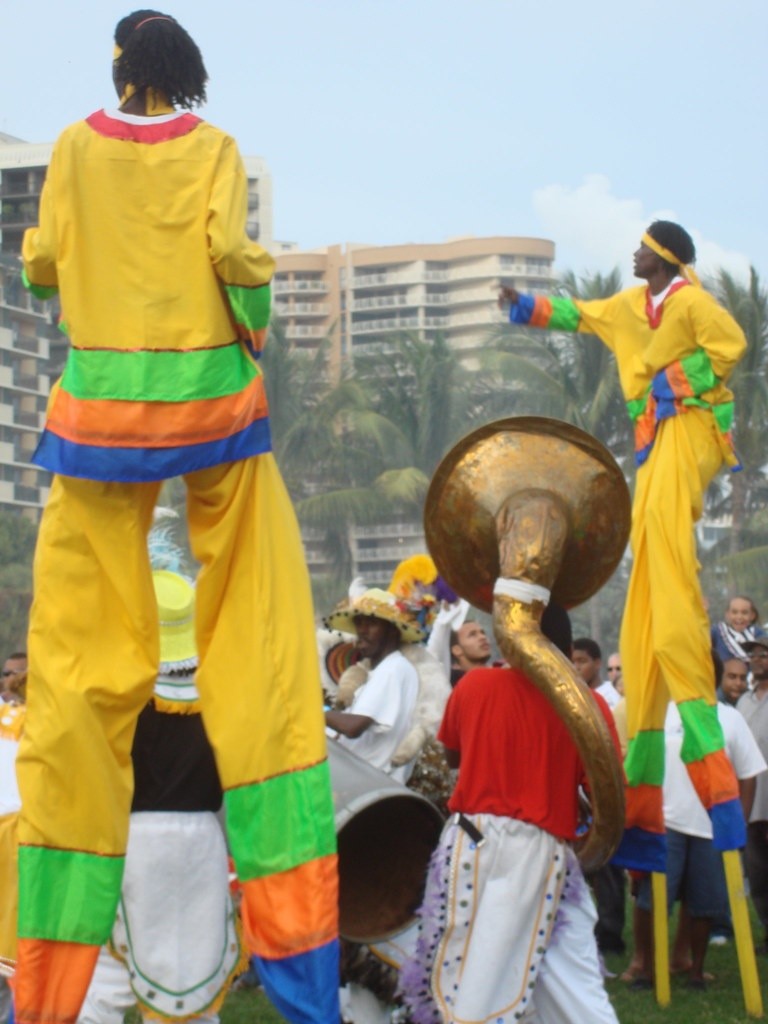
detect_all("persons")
[573,597,768,986]
[12,10,329,773]
[0,570,255,1024]
[497,222,748,694]
[317,554,504,1024]
[390,599,619,1024]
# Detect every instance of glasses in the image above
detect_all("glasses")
[2,671,11,676]
[607,666,619,672]
[748,651,768,658]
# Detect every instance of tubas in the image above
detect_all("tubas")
[423,416,628,874]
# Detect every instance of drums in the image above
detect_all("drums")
[321,729,451,940]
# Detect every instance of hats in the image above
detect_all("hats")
[741,638,768,652]
[148,571,199,672]
[323,587,427,641]
